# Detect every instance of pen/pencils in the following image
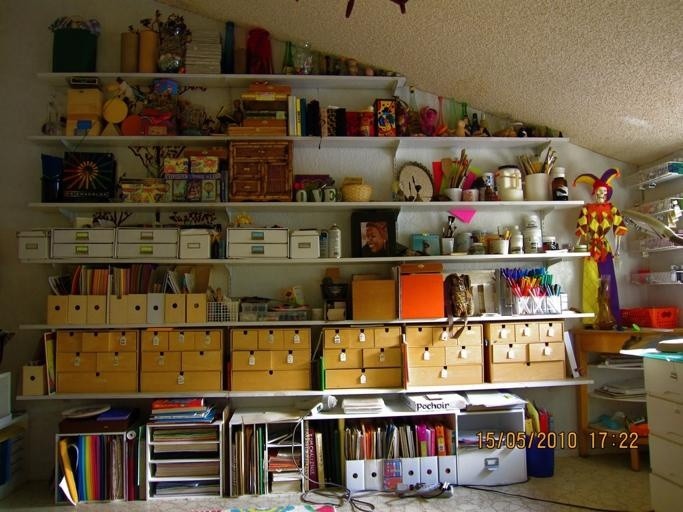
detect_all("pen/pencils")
[478,231,489,243]
[491,267,561,315]
[498,224,513,240]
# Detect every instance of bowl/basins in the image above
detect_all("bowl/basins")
[319,283,349,305]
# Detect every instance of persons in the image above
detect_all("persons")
[361,222,388,254]
[572,167,629,329]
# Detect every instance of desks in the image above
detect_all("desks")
[569,326,682,473]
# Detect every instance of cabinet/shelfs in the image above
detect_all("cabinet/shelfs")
[15,71,596,401]
[53,397,531,506]
[642,348,682,511]
[620,162,682,288]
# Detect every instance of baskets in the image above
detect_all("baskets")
[620,307,679,328]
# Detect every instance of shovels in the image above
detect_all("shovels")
[441,157,452,188]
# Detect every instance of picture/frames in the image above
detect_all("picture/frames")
[350,209,401,257]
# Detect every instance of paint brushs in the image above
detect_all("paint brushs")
[517,139,559,176]
[450,148,473,188]
[442,216,458,238]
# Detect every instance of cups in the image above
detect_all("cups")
[548,129,559,137]
[489,239,510,254]
[310,188,322,202]
[471,241,485,255]
[323,186,337,202]
[439,237,454,255]
[463,188,480,202]
[295,189,307,203]
[454,232,478,252]
[520,172,549,201]
[534,125,546,137]
[442,187,463,200]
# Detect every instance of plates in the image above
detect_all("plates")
[59,404,111,419]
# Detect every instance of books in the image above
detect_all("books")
[562,330,646,404]
[36,70,414,210]
[46,263,196,327]
[48,390,529,506]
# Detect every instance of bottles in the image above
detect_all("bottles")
[657,191,683,212]
[220,20,237,75]
[670,264,682,283]
[311,308,323,320]
[479,172,494,201]
[509,224,525,254]
[549,166,568,201]
[519,127,527,137]
[318,229,328,258]
[658,158,682,175]
[404,87,470,137]
[484,183,493,201]
[479,113,487,129]
[244,26,272,74]
[278,40,359,77]
[470,113,479,135]
